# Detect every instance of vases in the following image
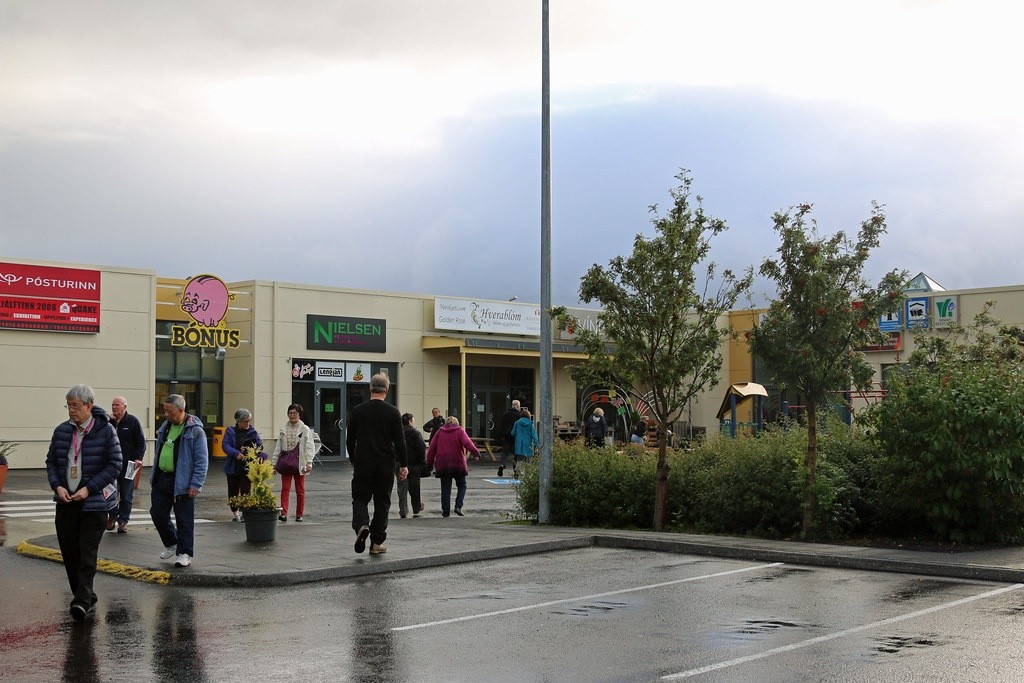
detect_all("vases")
[241,505,278,542]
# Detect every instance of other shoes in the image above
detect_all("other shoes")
[401,516,406,518]
[295,515,302,522]
[279,514,287,522]
[514,473,518,480]
[232,511,244,523]
[442,506,464,516]
[497,465,503,477]
[414,503,424,514]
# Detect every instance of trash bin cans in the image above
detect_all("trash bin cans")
[212,427,228,460]
[604,427,615,449]
[687,426,706,447]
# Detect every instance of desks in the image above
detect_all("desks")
[465,438,498,461]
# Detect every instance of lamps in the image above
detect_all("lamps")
[215,346,226,360]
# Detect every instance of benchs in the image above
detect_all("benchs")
[465,445,503,452]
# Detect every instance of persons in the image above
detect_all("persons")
[271,404,315,522]
[222,409,263,523]
[497,400,539,479]
[423,408,445,475]
[149,394,209,567]
[628,416,650,448]
[46,384,123,632]
[346,373,409,554]
[426,416,480,518]
[395,413,426,518]
[106,395,147,534]
[585,407,609,449]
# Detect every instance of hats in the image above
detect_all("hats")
[641,416,649,421]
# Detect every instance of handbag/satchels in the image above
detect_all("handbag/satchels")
[604,437,612,449]
[275,441,300,475]
[421,462,431,478]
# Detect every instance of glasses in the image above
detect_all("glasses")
[288,412,299,416]
[64,403,85,409]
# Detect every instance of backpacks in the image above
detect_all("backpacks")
[235,423,257,452]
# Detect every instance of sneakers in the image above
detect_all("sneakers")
[106,515,117,531]
[369,543,386,555]
[160,546,176,559]
[70,600,96,620]
[118,520,127,533]
[175,553,192,567]
[354,525,370,553]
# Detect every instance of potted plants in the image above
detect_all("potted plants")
[0,440,21,495]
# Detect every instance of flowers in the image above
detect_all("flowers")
[228,444,279,509]
